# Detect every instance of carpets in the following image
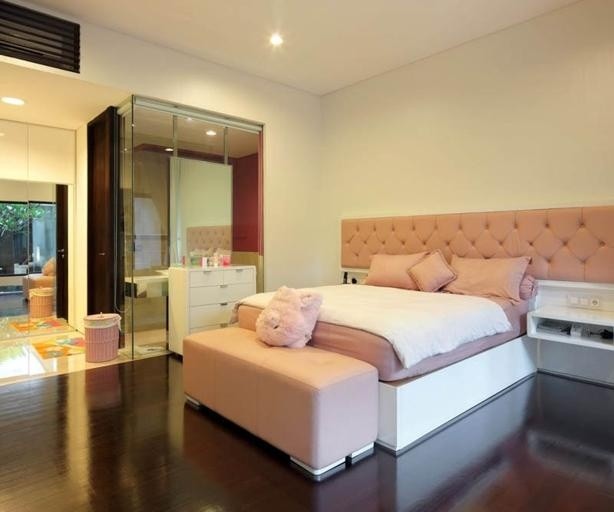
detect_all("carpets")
[32,336,86,360]
[9,315,67,333]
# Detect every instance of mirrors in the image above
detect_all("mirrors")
[0,178,68,342]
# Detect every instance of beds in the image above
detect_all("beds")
[229,250,546,459]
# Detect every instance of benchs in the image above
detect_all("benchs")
[181,327,380,475]
[22,274,56,304]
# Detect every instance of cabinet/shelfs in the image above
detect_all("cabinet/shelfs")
[167,264,257,356]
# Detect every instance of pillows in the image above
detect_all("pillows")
[253,285,322,350]
[519,274,538,299]
[362,252,427,291]
[443,253,532,306]
[407,251,457,295]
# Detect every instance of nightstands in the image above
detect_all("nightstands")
[526,305,613,352]
